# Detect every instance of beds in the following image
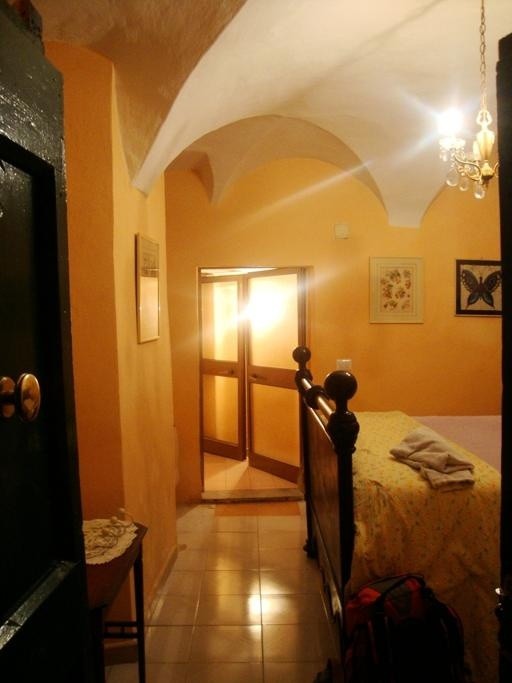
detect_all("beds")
[286,349,502,682]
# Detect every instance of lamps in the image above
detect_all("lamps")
[434,0,499,200]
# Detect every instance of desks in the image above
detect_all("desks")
[67,517,149,683]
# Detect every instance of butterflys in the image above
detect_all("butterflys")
[461,267,502,310]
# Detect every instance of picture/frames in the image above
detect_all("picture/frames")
[135,233,163,346]
[455,258,502,316]
[366,254,426,327]
